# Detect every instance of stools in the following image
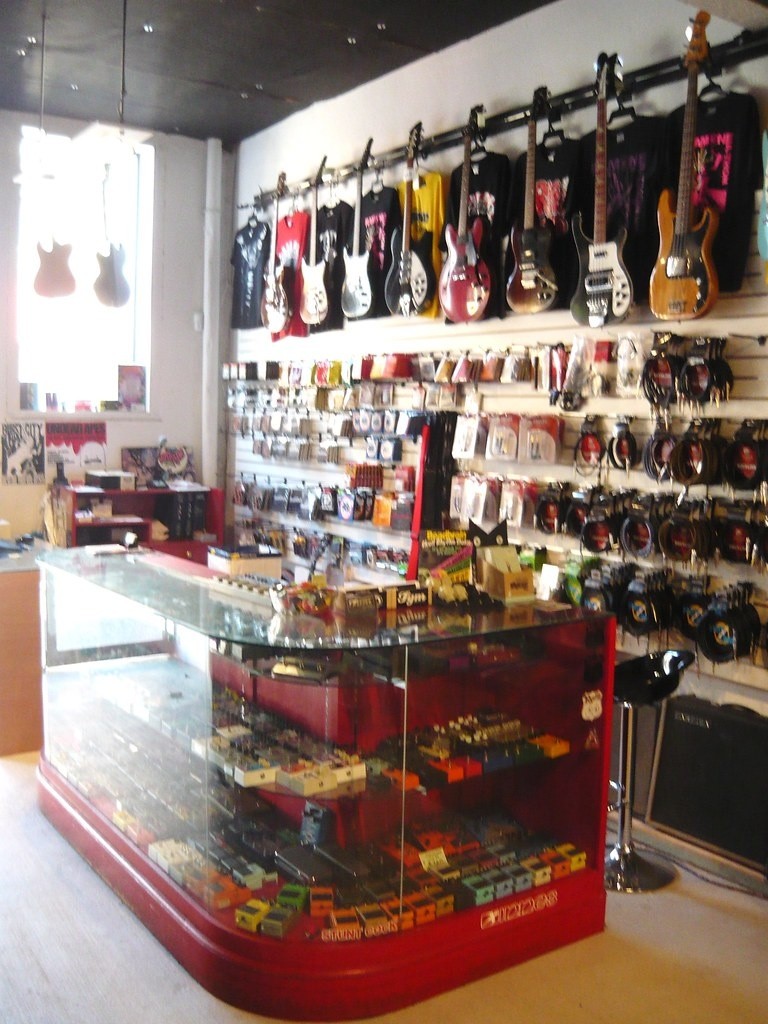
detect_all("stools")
[606,649,695,892]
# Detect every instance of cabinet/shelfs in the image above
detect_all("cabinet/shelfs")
[0,485,616,1023]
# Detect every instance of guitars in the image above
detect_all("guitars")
[258,7,727,337]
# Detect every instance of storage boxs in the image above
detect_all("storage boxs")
[321,545,535,613]
[208,544,281,580]
[86,472,136,488]
